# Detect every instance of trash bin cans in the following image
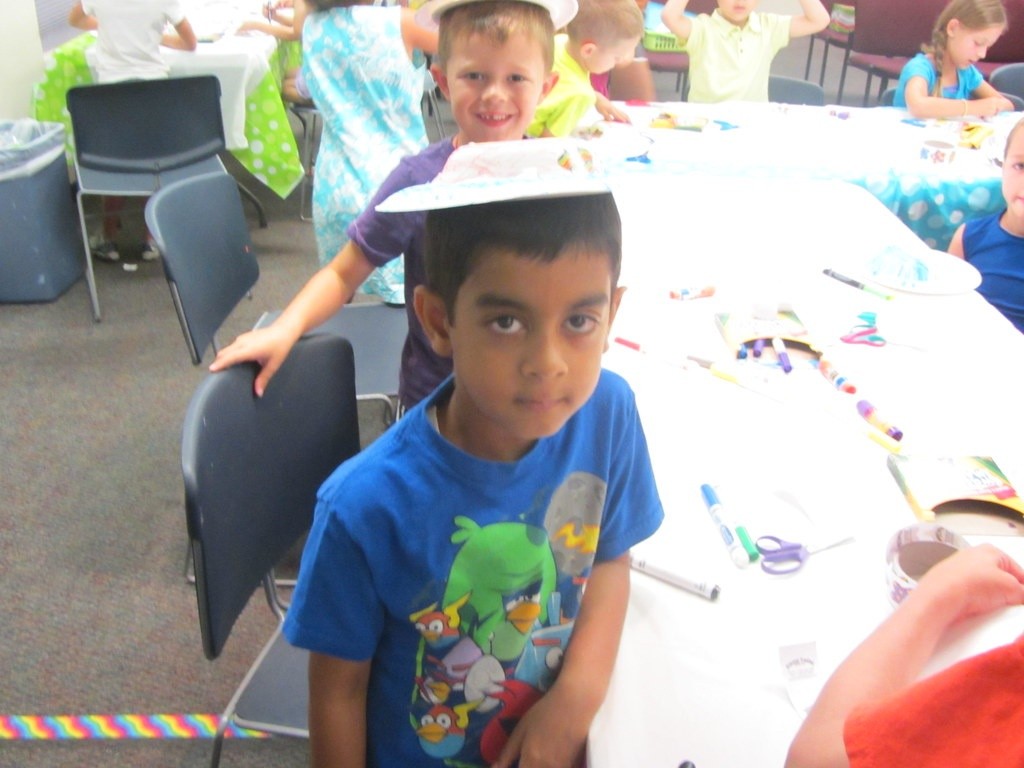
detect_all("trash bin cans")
[0,119,87,303]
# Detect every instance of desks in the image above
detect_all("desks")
[28,7,305,226]
[573,100,1024,767]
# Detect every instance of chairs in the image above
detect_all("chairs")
[65,75,230,321]
[143,171,411,588]
[183,333,361,768]
[645,0,1024,111]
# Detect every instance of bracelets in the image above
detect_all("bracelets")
[961,98,968,119]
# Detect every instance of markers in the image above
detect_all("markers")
[629,556,718,602]
[701,484,758,569]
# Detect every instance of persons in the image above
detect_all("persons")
[236,0,313,104]
[69,1,197,262]
[945,117,1024,334]
[208,0,579,422]
[523,0,646,143]
[785,541,1024,767]
[282,142,660,768]
[300,0,438,305]
[660,0,832,103]
[892,0,1016,120]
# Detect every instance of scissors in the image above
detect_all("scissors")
[820,324,888,348]
[624,149,651,164]
[752,532,851,575]
[712,118,740,130]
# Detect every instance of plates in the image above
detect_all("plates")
[873,246,983,296]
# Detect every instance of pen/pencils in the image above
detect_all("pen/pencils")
[824,267,893,300]
[615,337,650,354]
[888,458,938,524]
[686,355,748,389]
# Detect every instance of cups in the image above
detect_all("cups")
[883,519,973,611]
